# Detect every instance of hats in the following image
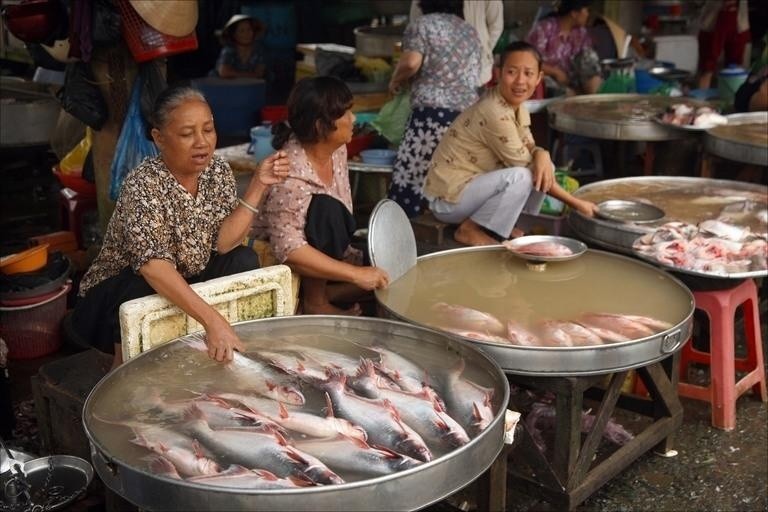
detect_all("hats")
[224,14,251,30]
[129,0,198,37]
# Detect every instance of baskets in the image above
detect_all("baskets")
[113,0,198,64]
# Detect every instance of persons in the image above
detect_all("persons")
[66,1,202,140]
[264,74,388,314]
[733,34,766,116]
[408,0,503,94]
[216,12,278,76]
[389,0,487,215]
[525,0,606,96]
[83,89,288,369]
[429,40,600,247]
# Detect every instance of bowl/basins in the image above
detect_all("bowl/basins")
[358,149,397,164]
[354,24,402,59]
[0,242,50,277]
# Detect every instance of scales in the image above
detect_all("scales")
[597,58,638,93]
[646,68,689,97]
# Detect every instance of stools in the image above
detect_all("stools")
[409,215,448,245]
[28,348,112,451]
[633,278,768,431]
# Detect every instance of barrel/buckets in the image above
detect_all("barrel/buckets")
[0,250,75,361]
[246,124,275,161]
[292,40,356,80]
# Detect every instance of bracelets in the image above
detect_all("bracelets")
[527,143,544,153]
[236,196,261,213]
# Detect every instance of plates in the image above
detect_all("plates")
[508,233,586,262]
[648,65,689,80]
[653,112,715,132]
[366,197,416,286]
[593,198,666,224]
[599,58,635,70]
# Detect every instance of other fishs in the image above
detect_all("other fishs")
[90,329,495,491]
